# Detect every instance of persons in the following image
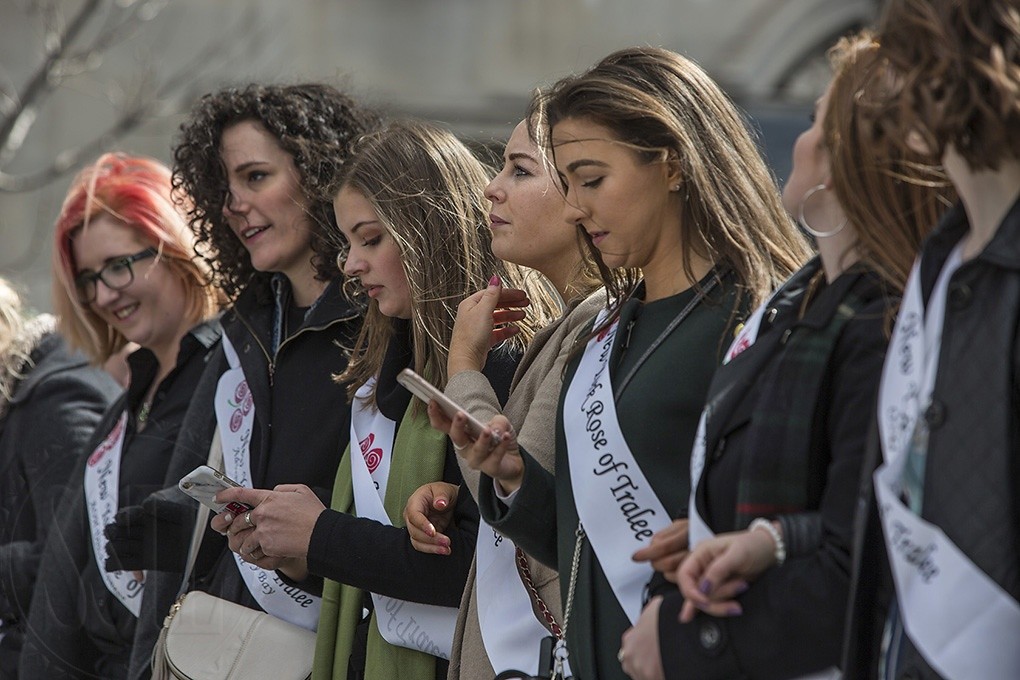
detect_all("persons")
[0,1,1020,680]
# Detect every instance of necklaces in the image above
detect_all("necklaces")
[135,401,150,422]
[282,290,292,340]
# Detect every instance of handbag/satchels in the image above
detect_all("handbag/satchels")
[151,591,317,680]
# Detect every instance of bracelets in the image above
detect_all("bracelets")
[747,517,786,566]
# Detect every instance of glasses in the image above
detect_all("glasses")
[72,246,159,305]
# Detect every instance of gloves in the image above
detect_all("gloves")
[103,496,229,580]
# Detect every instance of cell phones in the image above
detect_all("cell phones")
[394,366,504,450]
[177,463,255,517]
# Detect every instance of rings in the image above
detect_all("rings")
[452,436,471,450]
[245,510,254,528]
